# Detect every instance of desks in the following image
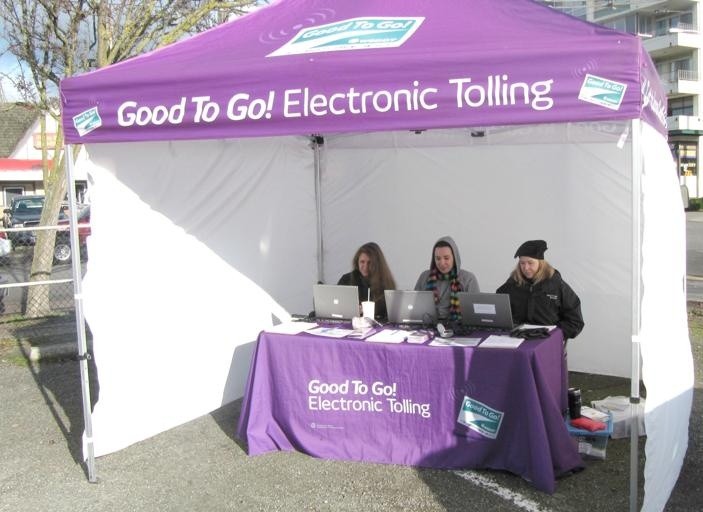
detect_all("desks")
[250,322,564,470]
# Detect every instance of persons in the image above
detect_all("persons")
[415,237,479,321]
[496,241,584,339]
[309,242,395,317]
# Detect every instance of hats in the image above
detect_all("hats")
[511,237,549,260]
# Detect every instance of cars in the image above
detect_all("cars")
[0,195,91,265]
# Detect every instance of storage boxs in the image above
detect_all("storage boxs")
[566,411,614,460]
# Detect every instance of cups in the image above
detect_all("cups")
[566,386,582,420]
[360,300,376,322]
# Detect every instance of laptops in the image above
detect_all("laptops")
[458,291,518,330]
[384,290,439,325]
[312,284,360,320]
